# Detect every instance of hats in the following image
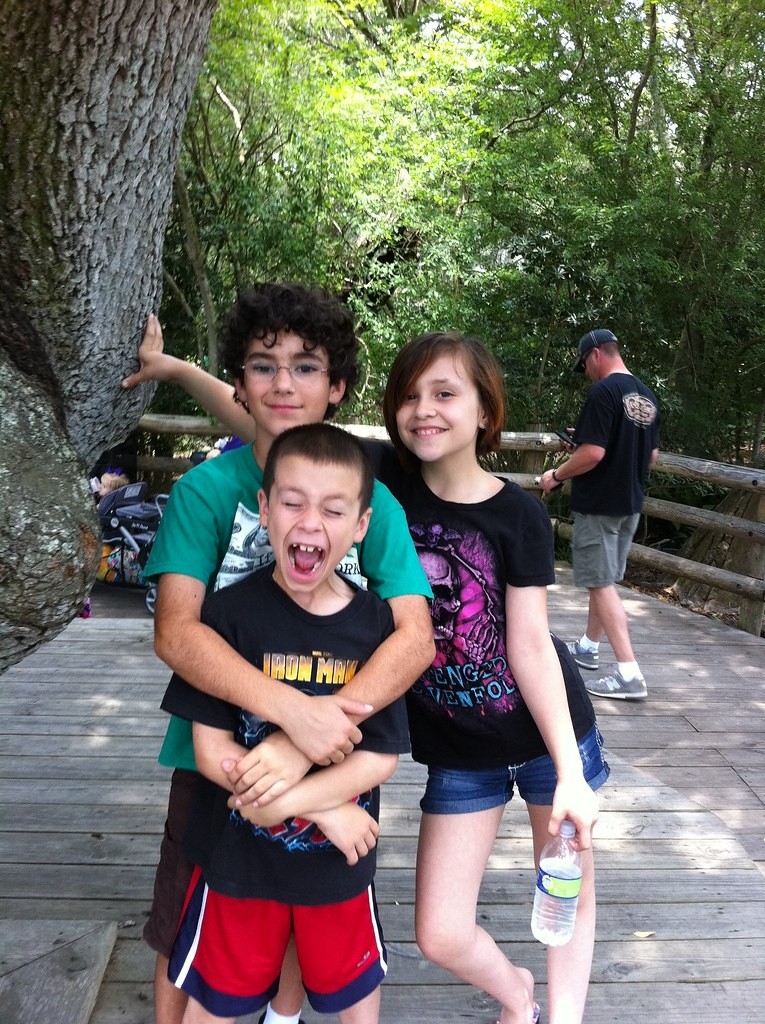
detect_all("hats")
[578,328,619,367]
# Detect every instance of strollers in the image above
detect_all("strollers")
[79,452,206,618]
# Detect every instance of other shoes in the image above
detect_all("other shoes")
[257,1009,305,1024]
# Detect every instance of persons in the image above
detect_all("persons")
[122,310,611,1024]
[158,420,414,1024]
[96,471,129,509]
[540,328,667,699]
[152,281,437,1024]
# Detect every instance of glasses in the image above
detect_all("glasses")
[240,359,334,384]
[581,347,594,369]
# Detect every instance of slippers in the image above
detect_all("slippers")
[496,1002,540,1024]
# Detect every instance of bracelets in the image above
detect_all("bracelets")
[552,469,566,482]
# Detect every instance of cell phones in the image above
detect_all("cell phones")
[555,429,577,447]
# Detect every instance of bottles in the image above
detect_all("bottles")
[530,820,582,946]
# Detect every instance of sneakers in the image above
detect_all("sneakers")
[565,640,598,670]
[584,666,648,700]
